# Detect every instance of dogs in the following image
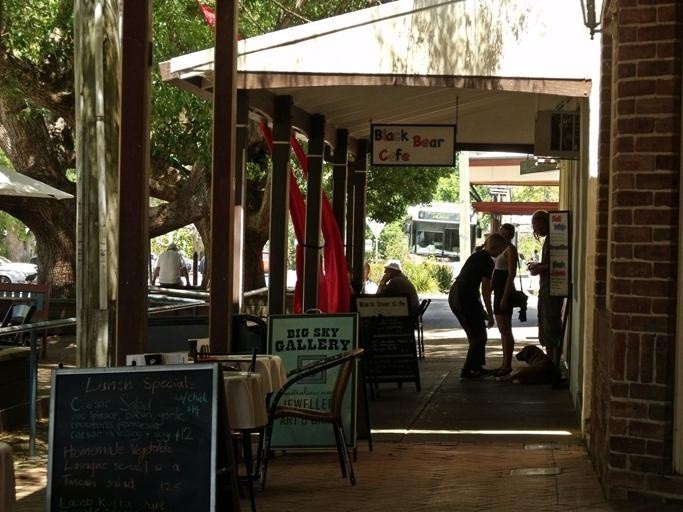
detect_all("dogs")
[485,345,562,386]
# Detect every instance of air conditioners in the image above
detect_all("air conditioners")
[533,111,579,160]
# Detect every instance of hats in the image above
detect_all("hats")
[384,263,402,273]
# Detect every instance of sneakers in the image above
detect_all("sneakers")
[461,367,513,379]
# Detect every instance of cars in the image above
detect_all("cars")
[0,255,39,287]
[147,253,190,278]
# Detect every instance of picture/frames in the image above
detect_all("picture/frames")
[548,211,572,299]
[352,295,422,401]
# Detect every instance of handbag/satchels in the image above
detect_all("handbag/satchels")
[515,291,527,307]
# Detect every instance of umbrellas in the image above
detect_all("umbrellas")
[0,166,75,201]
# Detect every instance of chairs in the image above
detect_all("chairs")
[192,312,365,498]
[413,298,431,358]
[0,302,36,348]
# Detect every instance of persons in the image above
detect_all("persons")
[449,234,506,378]
[364,262,378,295]
[520,250,540,271]
[376,263,420,324]
[490,224,518,376]
[152,244,190,287]
[526,210,563,360]
